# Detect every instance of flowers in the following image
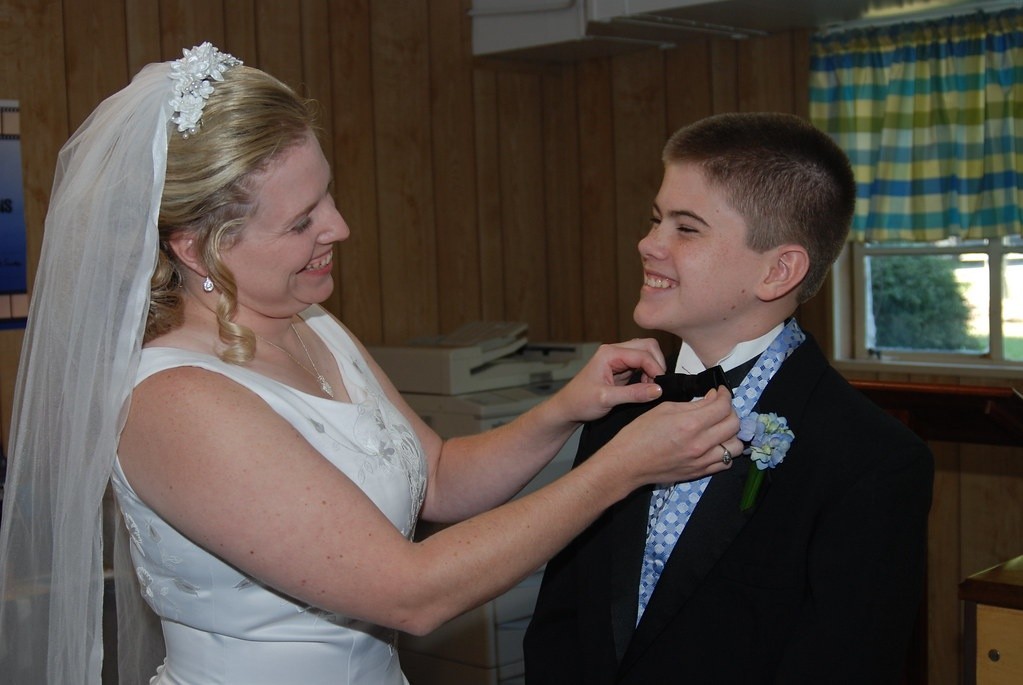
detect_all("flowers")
[736,409,796,521]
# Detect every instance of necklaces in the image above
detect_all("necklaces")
[176,284,335,399]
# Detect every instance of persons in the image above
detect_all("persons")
[523,108,933,684]
[108,64,745,684]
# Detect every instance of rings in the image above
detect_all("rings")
[718,443,732,465]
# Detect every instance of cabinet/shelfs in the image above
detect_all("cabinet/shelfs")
[470,1,867,60]
[955,555,1023,685]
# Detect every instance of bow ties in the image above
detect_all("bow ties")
[643,352,762,409]
